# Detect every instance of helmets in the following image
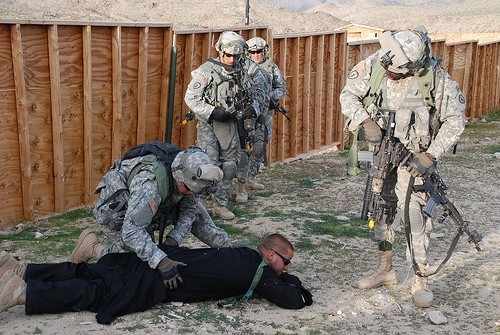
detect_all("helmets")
[384,30,427,73]
[220,31,246,55]
[172,149,214,192]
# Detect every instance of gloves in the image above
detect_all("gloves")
[212,106,229,123]
[242,106,255,118]
[158,259,187,291]
[164,237,178,247]
[406,154,434,177]
[362,122,382,144]
[269,102,274,110]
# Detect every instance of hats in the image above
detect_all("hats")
[246,37,267,51]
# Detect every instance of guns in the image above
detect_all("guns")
[391,142,484,252]
[268,96,292,121]
[224,70,251,150]
[360,109,397,221]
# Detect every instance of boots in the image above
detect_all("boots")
[213,200,235,220]
[0,270,27,313]
[357,250,397,290]
[72,229,105,264]
[411,264,433,308]
[249,175,264,190]
[236,182,248,203]
[0,251,27,280]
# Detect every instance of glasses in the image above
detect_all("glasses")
[270,248,290,265]
[224,53,239,57]
[250,49,263,54]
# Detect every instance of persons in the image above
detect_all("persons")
[340,27,467,308]
[234,38,285,204]
[1,234,312,326]
[71,140,237,279]
[182,29,259,219]
